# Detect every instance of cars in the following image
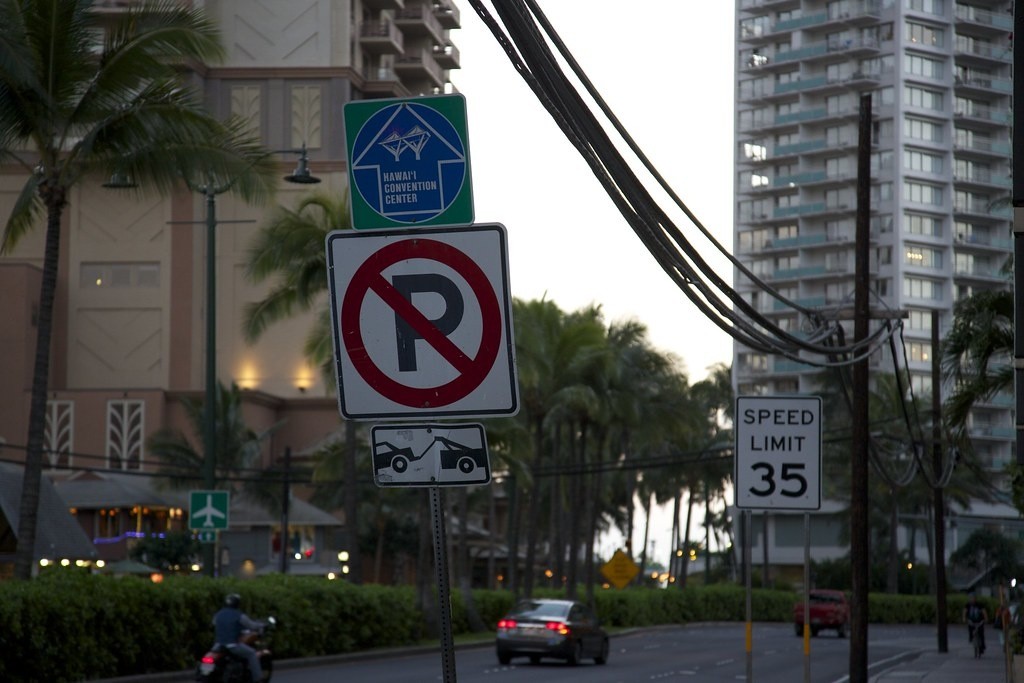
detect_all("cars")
[495,598,608,666]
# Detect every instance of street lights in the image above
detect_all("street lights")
[102,140,322,573]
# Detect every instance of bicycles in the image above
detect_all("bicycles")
[962,618,990,659]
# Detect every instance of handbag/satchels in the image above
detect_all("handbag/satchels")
[993,614,1002,629]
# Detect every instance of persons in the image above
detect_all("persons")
[963,595,988,653]
[996,603,1018,651]
[212,594,266,683]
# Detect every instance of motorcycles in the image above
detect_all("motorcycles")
[196,615,275,683]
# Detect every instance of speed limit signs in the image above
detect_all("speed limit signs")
[733,395,821,510]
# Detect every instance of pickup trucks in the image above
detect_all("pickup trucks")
[794,589,852,637]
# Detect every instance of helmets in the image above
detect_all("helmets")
[226,594,241,609]
